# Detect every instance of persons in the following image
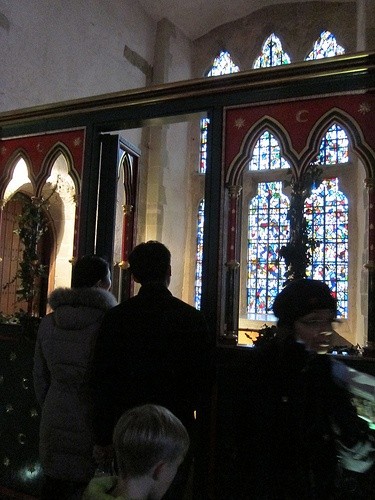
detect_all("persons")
[31,253,119,499]
[91,240,218,500]
[81,404,190,500]
[212,277,364,500]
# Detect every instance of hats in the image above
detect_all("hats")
[272,279,338,321]
